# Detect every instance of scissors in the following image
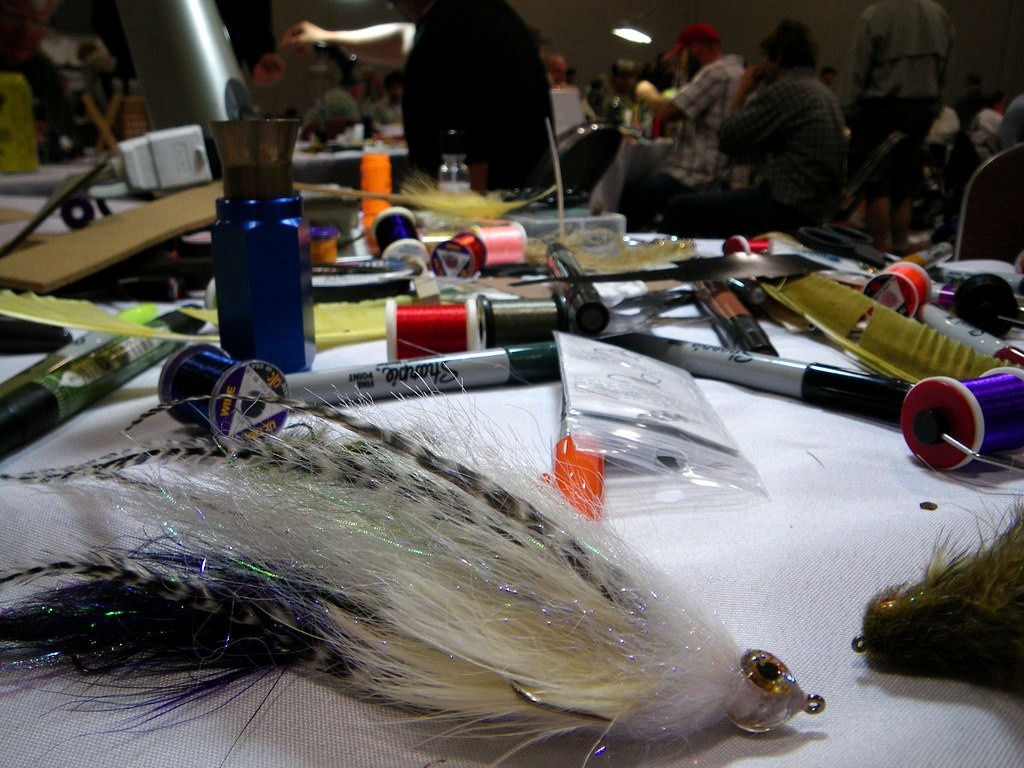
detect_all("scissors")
[797,220,890,269]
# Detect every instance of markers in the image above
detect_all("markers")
[543,242,610,335]
[691,279,780,356]
[902,242,953,268]
[918,282,1024,367]
[0,304,207,455]
[612,331,914,426]
[284,343,566,409]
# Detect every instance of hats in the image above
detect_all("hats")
[383,72,401,86]
[662,22,720,62]
[611,58,636,75]
[546,54,567,71]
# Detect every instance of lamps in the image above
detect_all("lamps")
[610,11,653,44]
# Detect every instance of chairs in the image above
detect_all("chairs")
[787,130,904,230]
[525,118,623,210]
[953,140,1023,265]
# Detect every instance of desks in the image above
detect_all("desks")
[0,143,1024,768]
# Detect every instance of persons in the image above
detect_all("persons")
[282,0,550,202]
[300,67,403,134]
[543,52,676,126]
[836,0,955,256]
[618,23,757,234]
[819,53,1024,246]
[663,17,844,239]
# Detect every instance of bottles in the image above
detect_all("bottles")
[439,154,471,195]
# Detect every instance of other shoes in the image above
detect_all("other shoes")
[848,210,865,228]
[878,238,909,252]
